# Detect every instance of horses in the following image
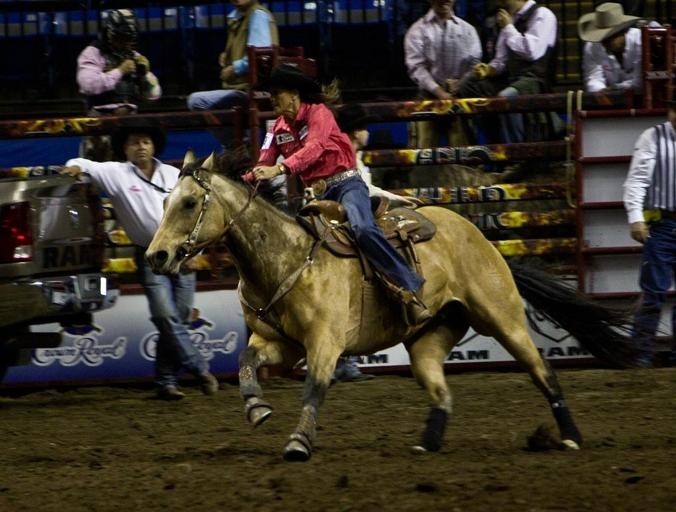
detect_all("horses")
[142,149,676,461]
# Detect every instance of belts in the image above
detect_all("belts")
[313,169,361,196]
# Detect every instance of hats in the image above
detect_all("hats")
[252,63,321,95]
[111,118,163,157]
[578,2,642,43]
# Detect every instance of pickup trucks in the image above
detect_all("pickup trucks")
[0,171,121,385]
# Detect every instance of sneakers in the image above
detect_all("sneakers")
[340,364,376,382]
[407,300,433,325]
[498,162,529,183]
[195,370,218,395]
[157,383,185,400]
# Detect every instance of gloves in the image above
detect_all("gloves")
[119,60,136,75]
[133,55,150,75]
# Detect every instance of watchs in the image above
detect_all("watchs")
[276,165,285,175]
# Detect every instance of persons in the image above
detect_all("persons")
[402,1,483,150]
[622,91,676,370]
[240,64,426,297]
[186,1,279,145]
[577,2,665,107]
[58,116,220,399]
[75,10,161,162]
[455,1,560,169]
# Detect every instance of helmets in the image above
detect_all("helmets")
[96,8,138,50]
